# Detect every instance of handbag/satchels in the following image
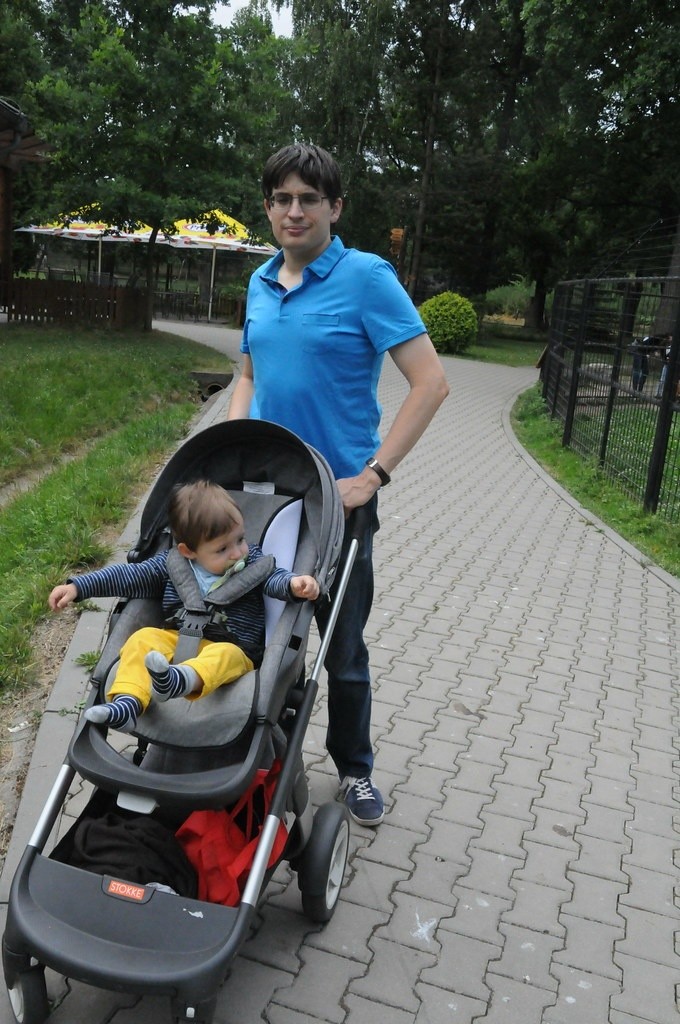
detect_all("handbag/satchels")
[175,769,289,908]
[72,811,198,900]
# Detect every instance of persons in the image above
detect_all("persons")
[227,144,450,826]
[48,482,320,734]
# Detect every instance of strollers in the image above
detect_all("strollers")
[0,418,365,1023]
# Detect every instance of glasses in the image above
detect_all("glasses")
[269,192,329,211]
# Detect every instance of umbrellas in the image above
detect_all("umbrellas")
[14,201,280,322]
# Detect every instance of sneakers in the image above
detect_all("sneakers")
[334,768,385,826]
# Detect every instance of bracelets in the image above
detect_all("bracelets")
[366,458,391,487]
